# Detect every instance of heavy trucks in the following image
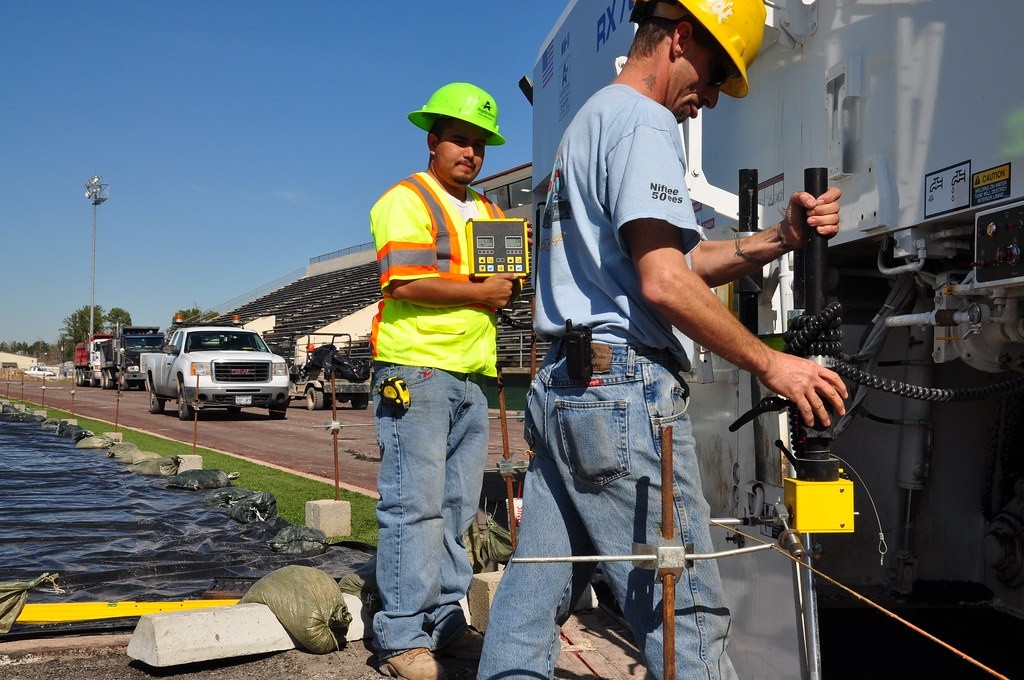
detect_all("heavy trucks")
[74,333,116,387]
[100,324,166,390]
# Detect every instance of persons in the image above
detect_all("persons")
[475,0,847,680]
[368,82,536,679]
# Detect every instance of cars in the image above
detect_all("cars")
[23,366,55,376]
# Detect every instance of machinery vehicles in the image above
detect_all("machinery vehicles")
[284,333,372,411]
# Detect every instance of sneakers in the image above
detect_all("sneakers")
[428,626,484,660]
[379,644,447,680]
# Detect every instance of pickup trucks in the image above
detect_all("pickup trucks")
[139,313,290,418]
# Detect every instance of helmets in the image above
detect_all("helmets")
[306,344,314,351]
[629,0,767,99]
[408,83,506,146]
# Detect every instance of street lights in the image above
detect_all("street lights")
[81,173,110,335]
